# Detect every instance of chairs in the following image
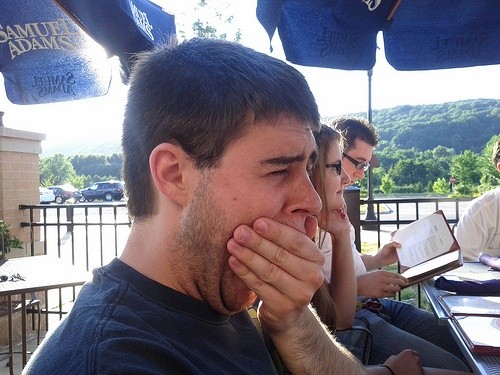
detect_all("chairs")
[0,292,41,367]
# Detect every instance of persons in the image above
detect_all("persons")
[307,119,477,375]
[21,35,372,375]
[455,138,500,261]
[315,114,469,366]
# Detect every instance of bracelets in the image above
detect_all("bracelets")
[378,364,395,375]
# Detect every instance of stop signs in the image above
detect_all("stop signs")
[449,176,455,184]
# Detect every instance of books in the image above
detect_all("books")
[388,209,464,289]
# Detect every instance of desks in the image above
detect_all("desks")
[0,254,89,375]
[418,260,500,375]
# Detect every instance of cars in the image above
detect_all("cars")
[38,186,54,206]
[78,180,125,201]
[45,184,81,205]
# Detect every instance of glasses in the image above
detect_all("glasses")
[344,153,372,172]
[314,156,343,175]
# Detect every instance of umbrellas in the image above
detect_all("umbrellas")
[0,0,176,105]
[256,0,500,70]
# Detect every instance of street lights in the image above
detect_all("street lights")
[361,39,383,232]
